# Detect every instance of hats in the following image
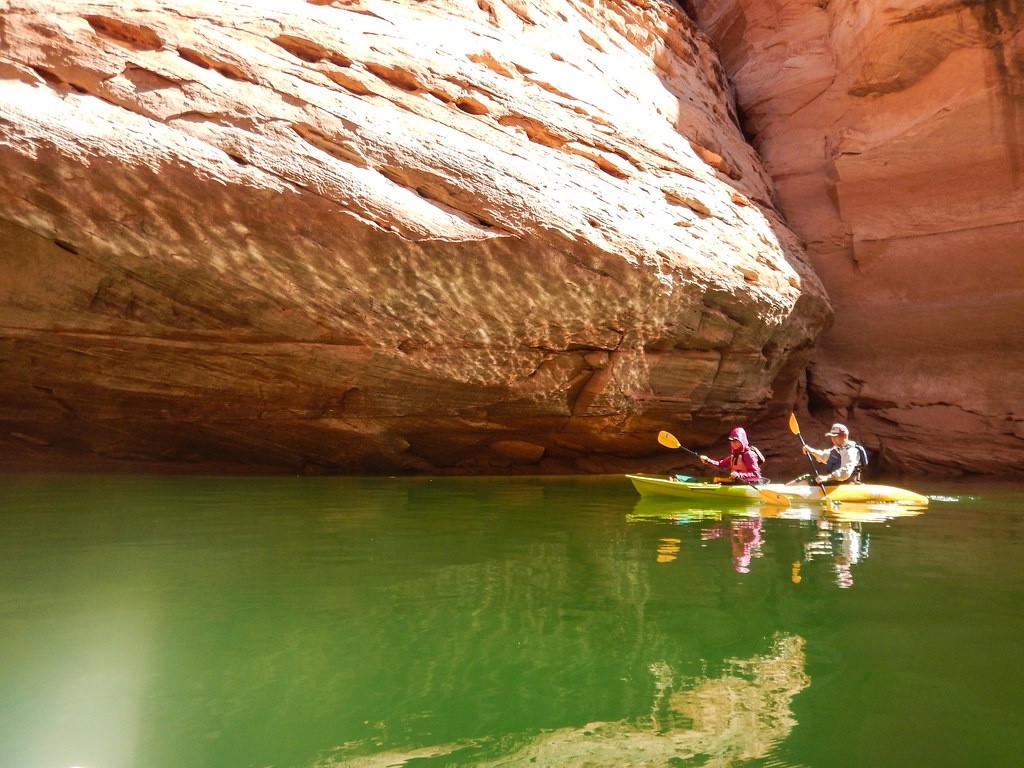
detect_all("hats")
[825,423,849,438]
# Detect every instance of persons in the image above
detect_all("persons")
[784,423,869,487]
[668,427,765,485]
[791,521,871,589]
[700,515,766,574]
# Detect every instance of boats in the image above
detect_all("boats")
[625,472,930,507]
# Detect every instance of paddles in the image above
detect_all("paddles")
[789,412,832,505]
[657,430,792,507]
